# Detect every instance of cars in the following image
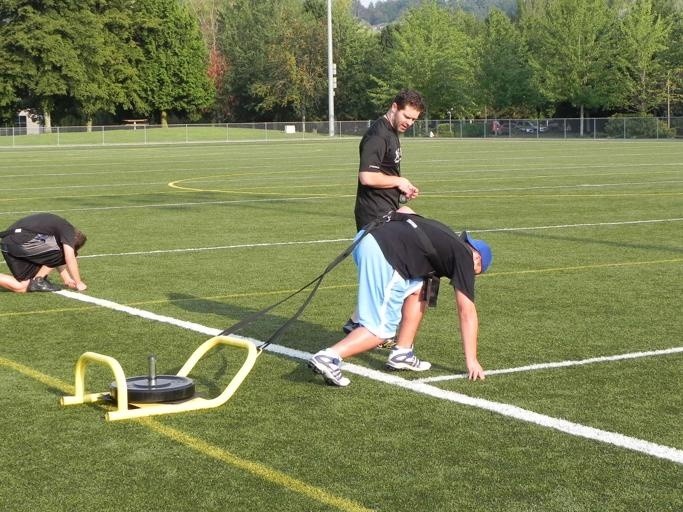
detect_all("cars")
[490,119,573,134]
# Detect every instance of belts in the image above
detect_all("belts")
[0,228,36,238]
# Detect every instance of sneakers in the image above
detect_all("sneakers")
[308,350,351,387]
[384,344,432,371]
[26,274,62,292]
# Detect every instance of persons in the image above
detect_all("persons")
[0,211,88,296]
[341,86,431,350]
[305,205,494,388]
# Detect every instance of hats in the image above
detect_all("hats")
[459,230,491,275]
